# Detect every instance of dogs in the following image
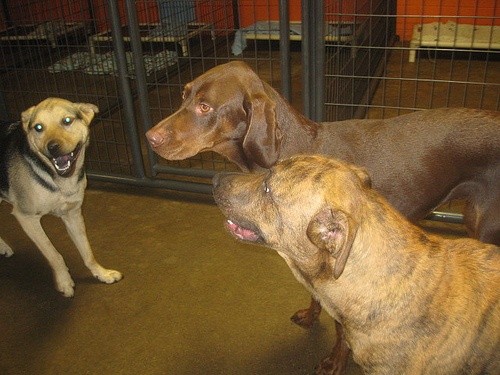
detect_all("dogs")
[0,96,124,298]
[209,153,500,375]
[143,59,499,374]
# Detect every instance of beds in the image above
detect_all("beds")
[232,20,368,56]
[0,22,88,58]
[90,22,215,56]
[409,20,500,62]
